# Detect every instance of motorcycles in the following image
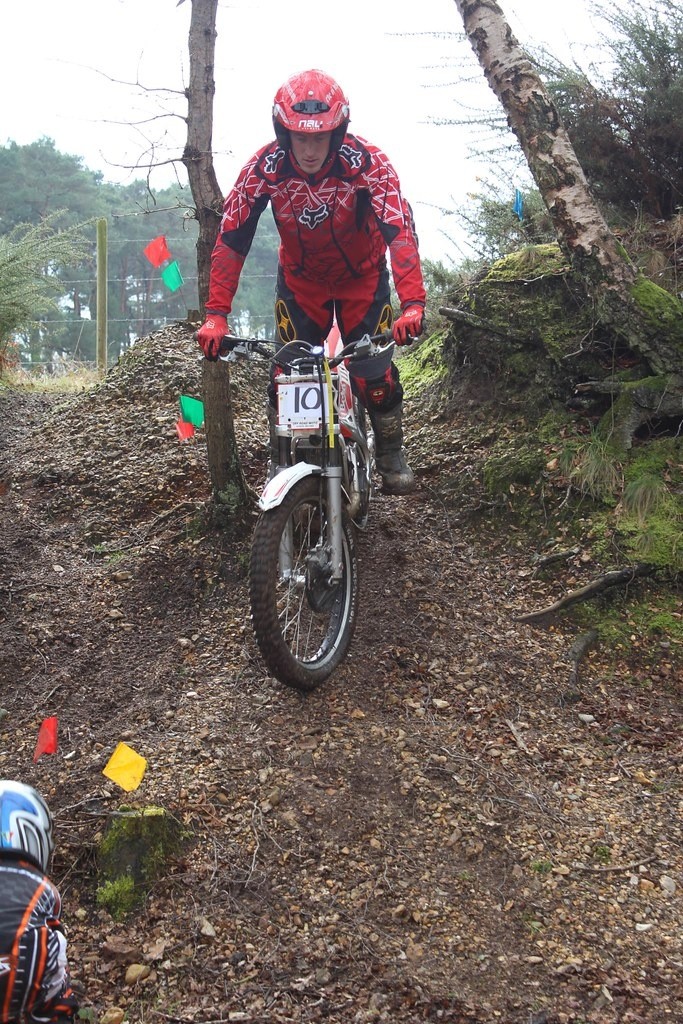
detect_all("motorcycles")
[191,300,429,696]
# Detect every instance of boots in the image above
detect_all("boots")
[367,402,415,495]
[265,403,279,488]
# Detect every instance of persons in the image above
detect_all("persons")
[195,68,426,496]
[0,776,81,1024]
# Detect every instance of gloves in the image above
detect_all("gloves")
[196,313,232,362]
[393,304,425,346]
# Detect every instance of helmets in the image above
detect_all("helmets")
[0,778,53,873]
[272,69,351,152]
